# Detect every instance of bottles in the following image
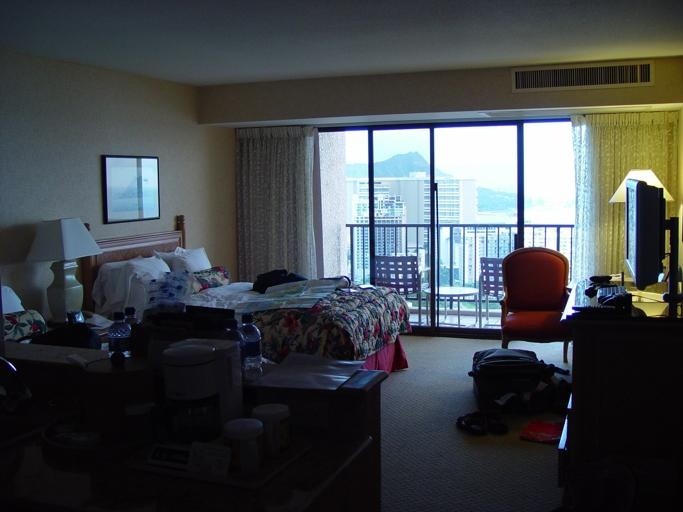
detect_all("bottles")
[224,313,262,371]
[106,304,138,360]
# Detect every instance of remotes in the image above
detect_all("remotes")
[573,305,618,313]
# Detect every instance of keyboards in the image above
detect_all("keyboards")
[596,286,627,304]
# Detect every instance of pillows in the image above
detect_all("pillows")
[0,283,25,315]
[101,246,231,310]
[2,309,49,343]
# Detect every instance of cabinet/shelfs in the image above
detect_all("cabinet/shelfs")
[555,278,682,511]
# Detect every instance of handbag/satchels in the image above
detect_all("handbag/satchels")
[249,268,296,294]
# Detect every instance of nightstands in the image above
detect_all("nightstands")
[48,312,114,344]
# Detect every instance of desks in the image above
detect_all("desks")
[424,286,479,328]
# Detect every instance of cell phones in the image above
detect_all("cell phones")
[589,274,613,282]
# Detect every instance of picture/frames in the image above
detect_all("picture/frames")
[100,153,161,223]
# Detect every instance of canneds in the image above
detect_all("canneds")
[222,418,264,478]
[253,403,290,468]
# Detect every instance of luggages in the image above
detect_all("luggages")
[467,345,553,415]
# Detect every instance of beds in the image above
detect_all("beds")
[77,215,411,373]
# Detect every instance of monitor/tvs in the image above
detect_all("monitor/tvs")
[625,179,683,304]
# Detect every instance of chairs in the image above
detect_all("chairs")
[375,255,430,325]
[498,247,572,363]
[478,257,504,328]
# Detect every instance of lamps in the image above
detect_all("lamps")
[25,216,104,322]
[609,167,674,203]
